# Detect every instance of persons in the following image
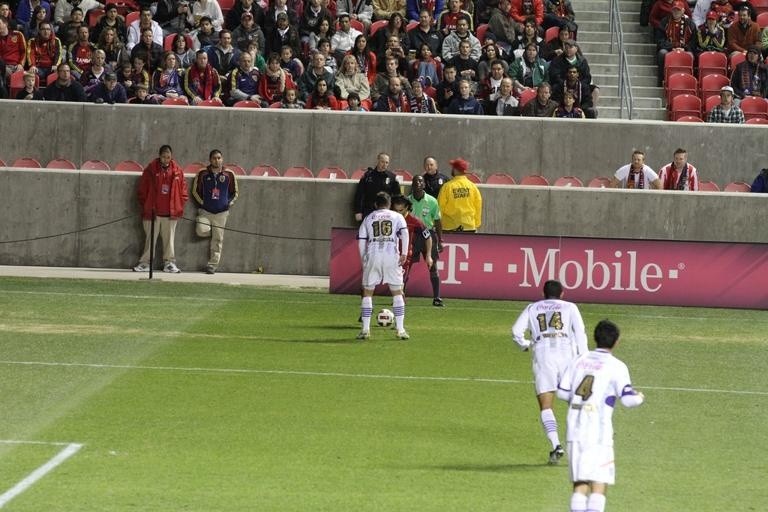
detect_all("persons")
[556,319,644,512]
[436,160,483,232]
[511,281,587,466]
[359,196,432,324]
[705,85,744,123]
[191,149,238,274]
[404,175,445,307]
[421,156,449,200]
[606,150,663,189]
[132,144,187,274]
[637,0,767,105]
[354,151,399,229]
[658,146,698,191]
[0,0,599,119]
[356,191,410,340]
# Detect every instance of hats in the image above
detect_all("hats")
[672,1,684,10]
[450,159,466,171]
[105,72,118,80]
[706,11,717,19]
[277,13,287,19]
[564,39,577,47]
[720,86,734,95]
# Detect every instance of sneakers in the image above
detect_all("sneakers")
[163,263,180,274]
[433,298,444,306]
[134,265,150,272]
[356,332,369,339]
[207,266,214,273]
[550,446,565,464]
[397,331,408,338]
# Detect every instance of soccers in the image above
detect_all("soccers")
[376,308,396,327]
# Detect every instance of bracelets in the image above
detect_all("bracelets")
[439,239,443,242]
[425,254,432,257]
